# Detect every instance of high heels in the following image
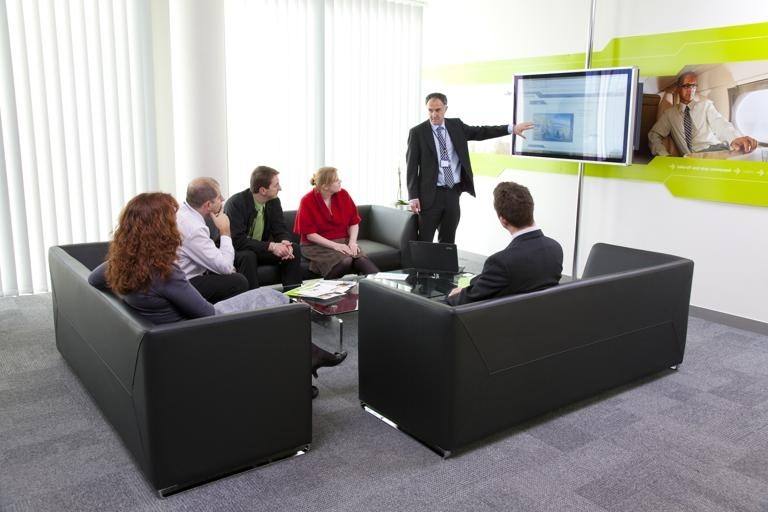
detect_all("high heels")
[312,350,348,379]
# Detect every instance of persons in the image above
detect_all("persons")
[170,177,249,304]
[405,93,535,296]
[442,180,564,309]
[293,168,381,276]
[87,194,349,399]
[213,166,301,286]
[647,69,759,164]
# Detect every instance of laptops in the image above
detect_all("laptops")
[407,241,465,274]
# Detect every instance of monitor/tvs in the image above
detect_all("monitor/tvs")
[510,66,639,166]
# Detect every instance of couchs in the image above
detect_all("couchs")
[44,240,312,496]
[356,239,693,458]
[206,204,418,282]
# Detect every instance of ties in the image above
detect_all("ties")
[683,105,693,152]
[436,131,455,190]
[252,208,265,245]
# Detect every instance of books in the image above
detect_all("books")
[283,277,358,300]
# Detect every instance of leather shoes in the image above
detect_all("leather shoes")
[312,385,319,399]
